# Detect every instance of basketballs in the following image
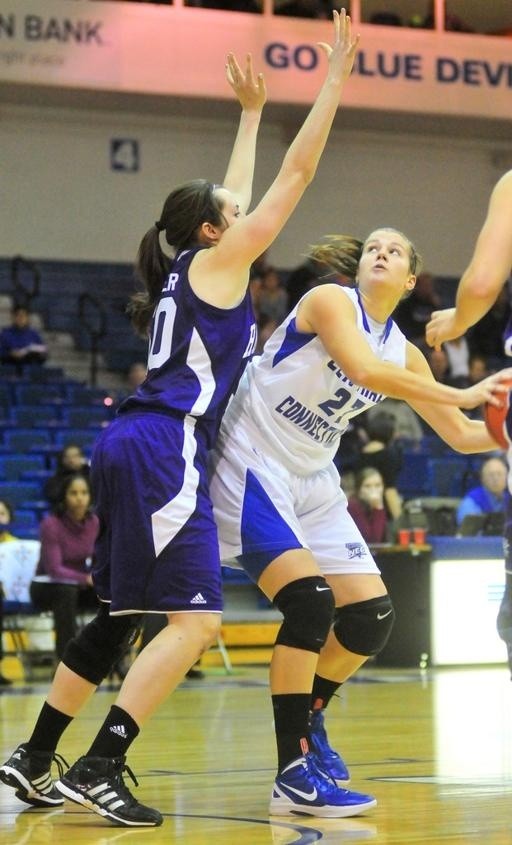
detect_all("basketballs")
[485,380,512,450]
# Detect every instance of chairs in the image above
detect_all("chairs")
[0,255,512,674]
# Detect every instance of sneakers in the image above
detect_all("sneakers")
[55,754,162,826]
[0,743,69,805]
[269,705,377,817]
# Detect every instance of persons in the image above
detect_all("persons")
[334,272,512,544]
[0,441,168,681]
[0,7,365,827]
[253,263,289,354]
[140,1,481,32]
[2,304,48,367]
[426,155,510,352]
[130,349,147,388]
[209,226,510,819]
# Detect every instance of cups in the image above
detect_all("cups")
[412,528,425,546]
[398,530,411,545]
